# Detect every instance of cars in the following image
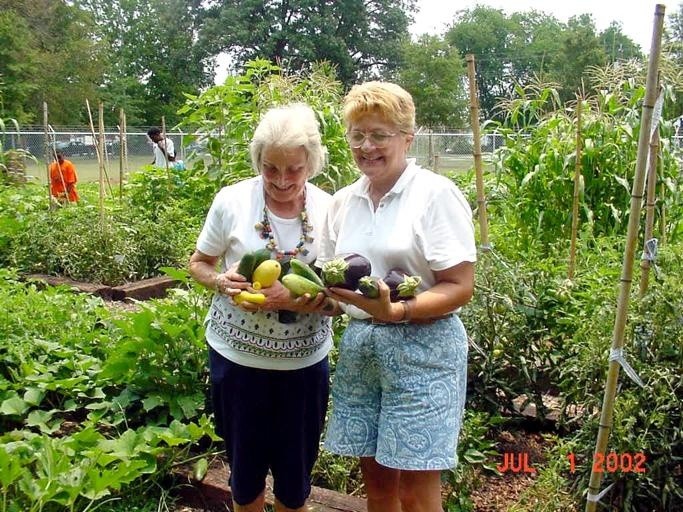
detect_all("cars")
[23,128,124,162]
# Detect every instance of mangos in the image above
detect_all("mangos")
[252,260,281,289]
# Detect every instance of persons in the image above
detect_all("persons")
[187,98,339,509]
[144,125,178,172]
[46,147,81,205]
[292,78,478,512]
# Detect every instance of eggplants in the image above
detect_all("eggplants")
[323,254,371,291]
[357,276,379,299]
[383,268,421,302]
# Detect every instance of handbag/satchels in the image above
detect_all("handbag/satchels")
[167,149,176,161]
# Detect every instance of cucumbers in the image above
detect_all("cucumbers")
[290,258,325,287]
[253,248,271,269]
[282,275,325,301]
[237,255,254,281]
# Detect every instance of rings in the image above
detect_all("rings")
[223,286,227,293]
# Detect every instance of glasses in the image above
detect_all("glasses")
[342,126,407,149]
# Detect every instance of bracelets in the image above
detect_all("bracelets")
[393,297,411,327]
[215,272,222,295]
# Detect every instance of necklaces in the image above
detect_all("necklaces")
[257,200,311,262]
